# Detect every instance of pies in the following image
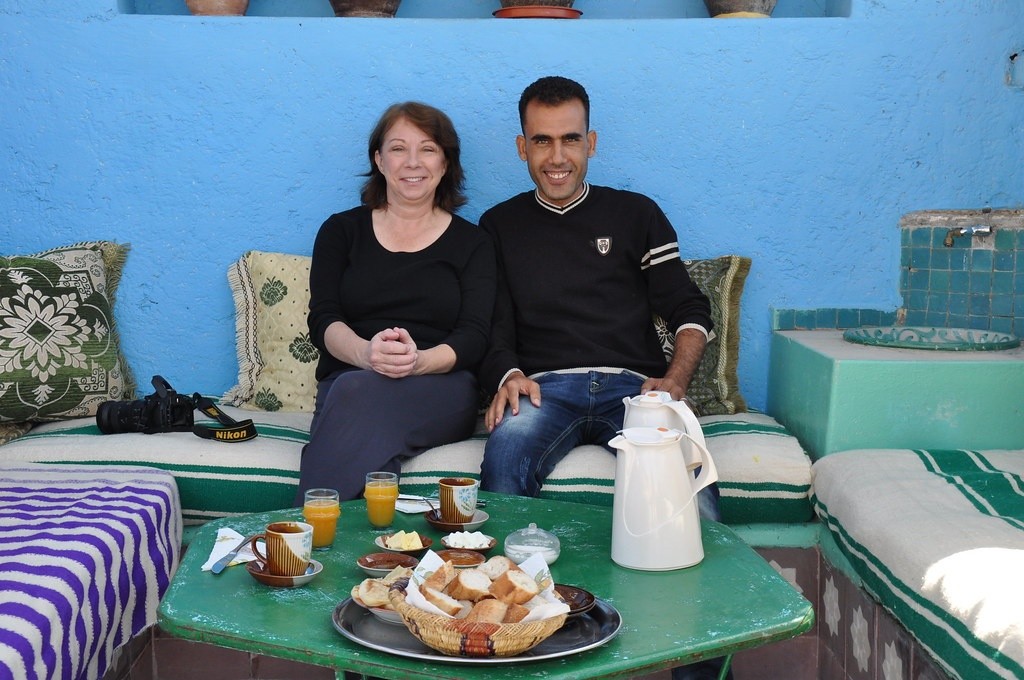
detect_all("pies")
[350,565,413,611]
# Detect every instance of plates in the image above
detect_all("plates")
[424,507,488,533]
[246,559,324,588]
[357,535,499,578]
[370,608,404,625]
[555,583,595,624]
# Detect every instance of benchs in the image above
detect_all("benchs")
[0,397,1024,680]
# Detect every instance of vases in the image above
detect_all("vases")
[186,0,790,19]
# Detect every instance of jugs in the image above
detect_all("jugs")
[608,427,719,571]
[623,391,707,482]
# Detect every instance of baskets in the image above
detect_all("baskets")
[389,576,570,655]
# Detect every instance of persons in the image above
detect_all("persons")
[292,102,497,508]
[478,76,721,522]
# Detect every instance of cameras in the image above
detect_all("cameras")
[97,389,195,435]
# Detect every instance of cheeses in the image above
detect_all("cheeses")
[385,530,423,549]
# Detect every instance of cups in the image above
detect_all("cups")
[439,477,479,523]
[365,471,399,529]
[302,489,341,550]
[251,521,314,576]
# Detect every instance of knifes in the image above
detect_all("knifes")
[211,535,255,574]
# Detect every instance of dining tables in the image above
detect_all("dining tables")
[170,494,817,679]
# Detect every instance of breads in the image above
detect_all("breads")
[420,555,540,622]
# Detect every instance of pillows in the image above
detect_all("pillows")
[0,237,128,435]
[226,251,323,411]
[642,255,744,422]
